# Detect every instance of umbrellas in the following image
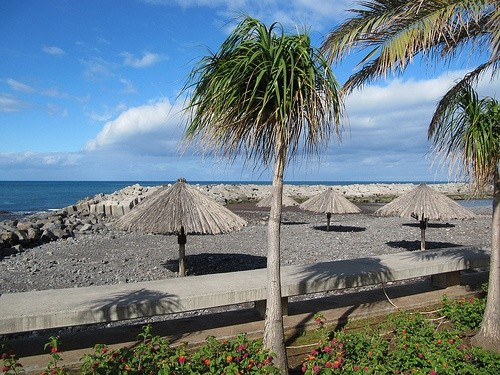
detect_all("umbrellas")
[298,185,363,232]
[109,178,248,281]
[255,189,301,210]
[374,180,476,252]
